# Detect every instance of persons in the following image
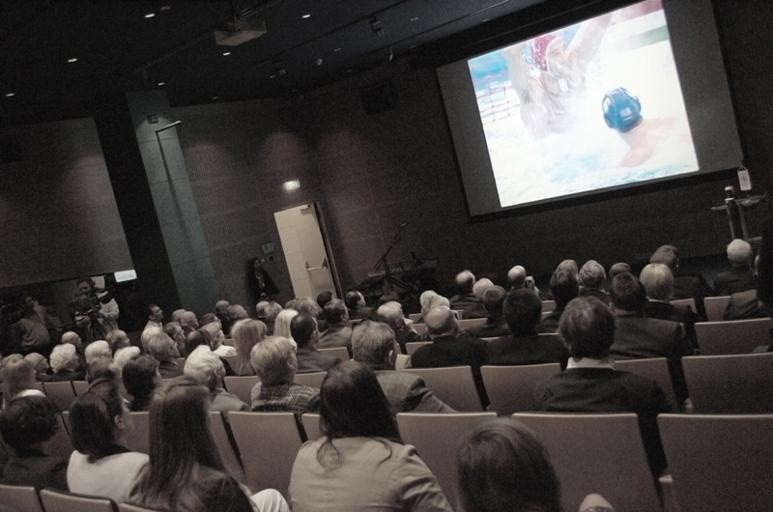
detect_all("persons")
[246,255,281,301]
[503,3,641,120]
[451,233,773,478]
[142,290,492,438]
[451,417,616,512]
[0,273,119,374]
[599,82,698,186]
[288,362,452,512]
[0,330,290,511]
